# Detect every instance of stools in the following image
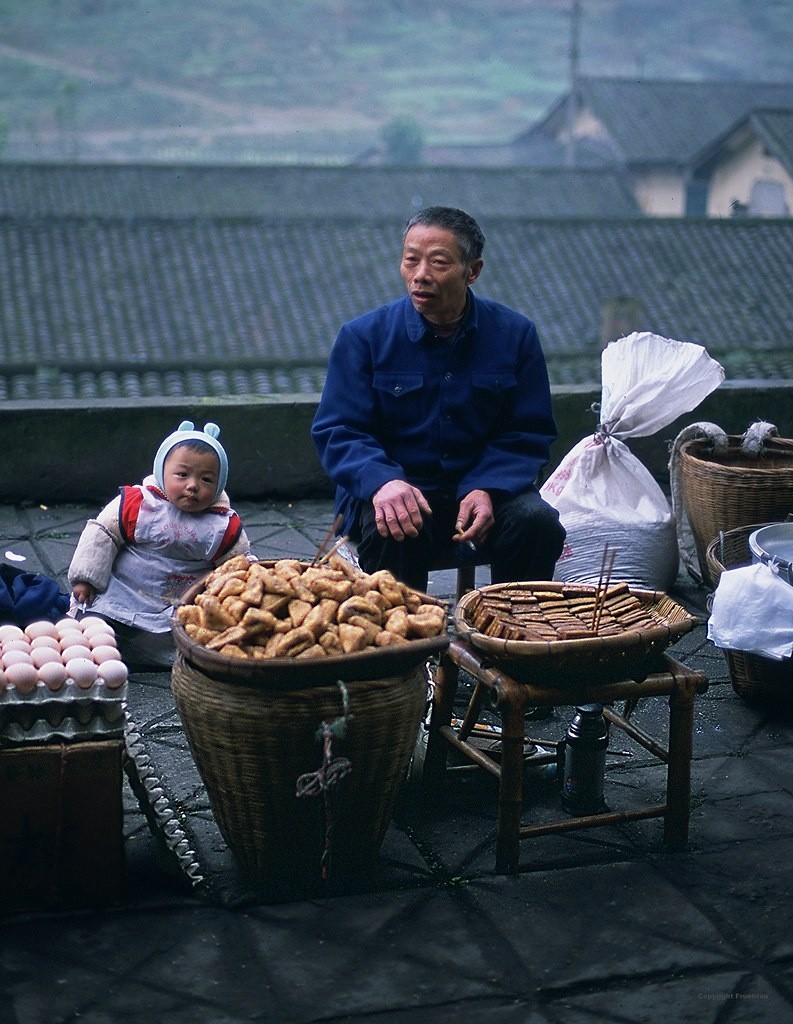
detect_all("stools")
[422,643,709,875]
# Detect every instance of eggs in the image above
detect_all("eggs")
[0,616,129,696]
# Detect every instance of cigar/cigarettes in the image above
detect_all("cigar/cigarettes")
[457,528,478,551]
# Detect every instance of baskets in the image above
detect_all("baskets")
[721,649,793,707]
[679,436,793,589]
[455,581,700,670]
[170,560,450,879]
[706,522,793,613]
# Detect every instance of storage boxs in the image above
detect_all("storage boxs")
[0,739,126,915]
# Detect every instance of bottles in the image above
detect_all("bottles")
[562,703,609,816]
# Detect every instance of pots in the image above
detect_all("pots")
[748,522,793,587]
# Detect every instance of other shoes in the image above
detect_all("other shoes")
[524,706,554,720]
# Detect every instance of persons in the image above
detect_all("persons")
[310,206,567,721]
[67,421,259,648]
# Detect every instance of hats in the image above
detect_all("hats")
[154,421,228,505]
